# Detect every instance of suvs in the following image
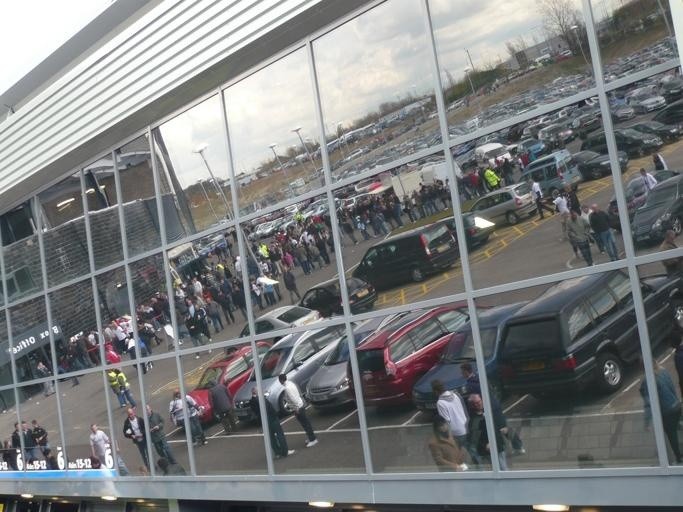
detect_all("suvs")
[346,302,497,412]
[497,267,682,406]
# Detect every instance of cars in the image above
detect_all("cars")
[298,276,378,316]
[628,173,682,249]
[170,342,282,425]
[434,210,495,249]
[222,304,326,357]
[194,114,390,259]
[305,309,410,411]
[411,298,531,418]
[233,318,361,425]
[247,36,682,244]
[605,170,679,231]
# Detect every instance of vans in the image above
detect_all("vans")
[349,220,459,295]
[466,180,538,229]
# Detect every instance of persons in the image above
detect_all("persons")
[0,124,682,471]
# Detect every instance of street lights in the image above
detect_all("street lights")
[569,25,590,70]
